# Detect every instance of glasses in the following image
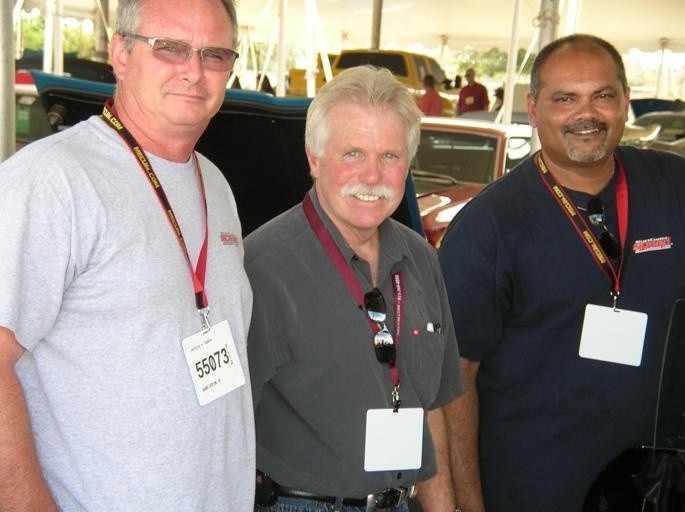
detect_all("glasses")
[363,286,396,366]
[587,197,619,259]
[120,31,239,72]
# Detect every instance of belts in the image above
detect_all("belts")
[274,485,414,511]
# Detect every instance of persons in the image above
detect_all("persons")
[2,0,255,512]
[436,34,683,509]
[245,65,468,511]
[456,68,489,117]
[417,74,444,117]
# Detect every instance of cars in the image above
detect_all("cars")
[331,48,463,117]
[410,117,534,252]
